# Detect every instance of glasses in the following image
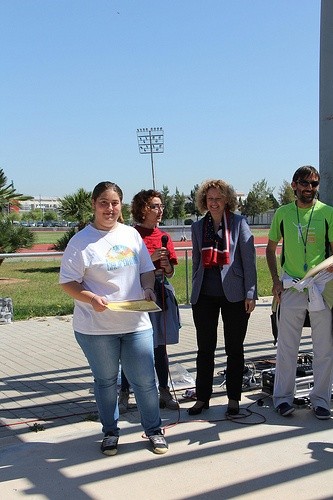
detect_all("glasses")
[296,179,320,188]
[149,204,165,210]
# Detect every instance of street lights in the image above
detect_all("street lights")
[136,127,165,191]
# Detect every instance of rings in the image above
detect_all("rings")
[251,308,253,309]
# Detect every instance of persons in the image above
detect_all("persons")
[265,165,333,418]
[188,178,259,416]
[58,181,183,456]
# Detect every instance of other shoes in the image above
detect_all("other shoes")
[117,390,129,413]
[314,406,331,419]
[277,402,295,416]
[227,399,239,415]
[189,400,209,415]
[159,387,179,410]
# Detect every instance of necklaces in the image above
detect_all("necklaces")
[95,226,115,248]
[297,200,316,272]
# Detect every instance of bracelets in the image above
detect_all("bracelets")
[164,265,174,274]
[272,274,279,280]
[144,288,154,293]
[90,294,96,304]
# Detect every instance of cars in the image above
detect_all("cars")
[12,220,79,227]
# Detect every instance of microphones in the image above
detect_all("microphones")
[161,235,169,248]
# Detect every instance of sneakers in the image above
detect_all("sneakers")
[100,431,119,456]
[149,433,168,454]
